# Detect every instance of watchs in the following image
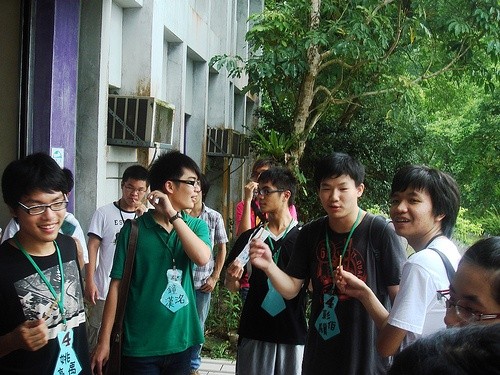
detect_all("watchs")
[169,212,182,223]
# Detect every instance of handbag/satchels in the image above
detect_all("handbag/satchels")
[94,328,121,375]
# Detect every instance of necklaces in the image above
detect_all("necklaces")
[118,200,136,224]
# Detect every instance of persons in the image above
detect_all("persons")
[1,168,89,264]
[0,152,92,375]
[84,165,150,356]
[249,153,408,375]
[235,159,297,308]
[437,236,500,328]
[335,166,462,358]
[89,151,212,375]
[224,169,308,375]
[185,174,229,375]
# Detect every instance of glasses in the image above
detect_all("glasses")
[436,290,500,325]
[169,179,201,188]
[15,193,69,216]
[124,183,148,194]
[253,189,286,195]
[251,171,263,178]
